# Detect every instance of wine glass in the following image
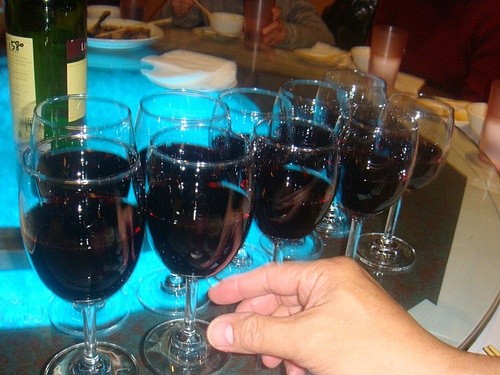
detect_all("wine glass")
[26,92,139,337]
[130,90,231,315]
[141,124,260,375]
[259,76,352,259]
[334,97,419,260]
[206,85,295,282]
[251,113,341,264]
[354,89,456,273]
[310,66,388,237]
[16,134,147,375]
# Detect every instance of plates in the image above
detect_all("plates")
[193,26,244,40]
[87,16,165,51]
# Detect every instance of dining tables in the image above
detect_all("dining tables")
[0,20,498,374]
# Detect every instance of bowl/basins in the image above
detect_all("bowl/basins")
[351,46,371,72]
[464,101,490,137]
[87,6,120,19]
[209,11,244,36]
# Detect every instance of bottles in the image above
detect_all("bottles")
[4,0,87,158]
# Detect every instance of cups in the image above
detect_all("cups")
[479,79,500,176]
[368,23,410,90]
[243,0,275,51]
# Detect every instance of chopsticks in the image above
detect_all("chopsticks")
[95,17,172,38]
[192,0,216,19]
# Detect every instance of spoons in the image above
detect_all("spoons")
[90,10,111,34]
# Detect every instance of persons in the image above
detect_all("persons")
[145,0,336,48]
[362,1,500,113]
[207,255,500,375]
